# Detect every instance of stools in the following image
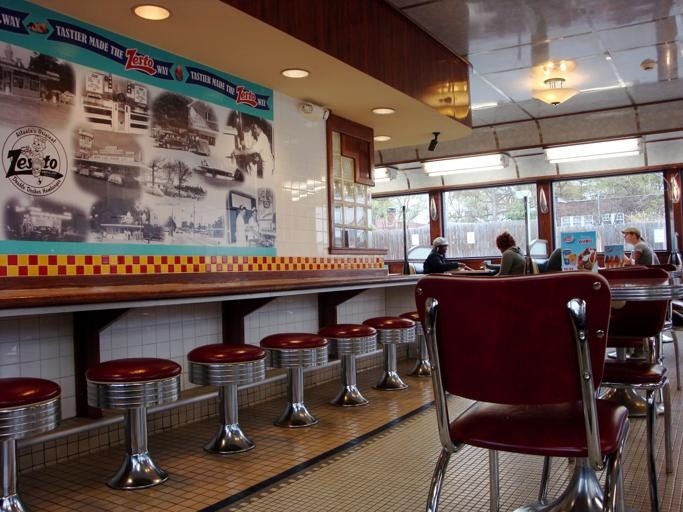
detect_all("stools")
[319,324,376,406]
[86,358,182,490]
[259,332,328,428]
[400,312,432,377]
[0,377,62,512]
[186,342,268,454]
[363,316,416,392]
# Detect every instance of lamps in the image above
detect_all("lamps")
[669,176,680,204]
[539,188,548,214]
[430,199,438,221]
[533,78,577,105]
[428,132,440,150]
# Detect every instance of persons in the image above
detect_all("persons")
[236,205,246,246]
[484,232,525,276]
[233,121,274,178]
[545,248,561,272]
[622,227,654,267]
[246,206,259,246]
[423,237,471,274]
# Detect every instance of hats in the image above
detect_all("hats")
[621,226,640,234]
[432,236,449,248]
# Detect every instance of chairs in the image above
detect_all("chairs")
[634,265,683,393]
[415,273,630,511]
[593,267,673,512]
